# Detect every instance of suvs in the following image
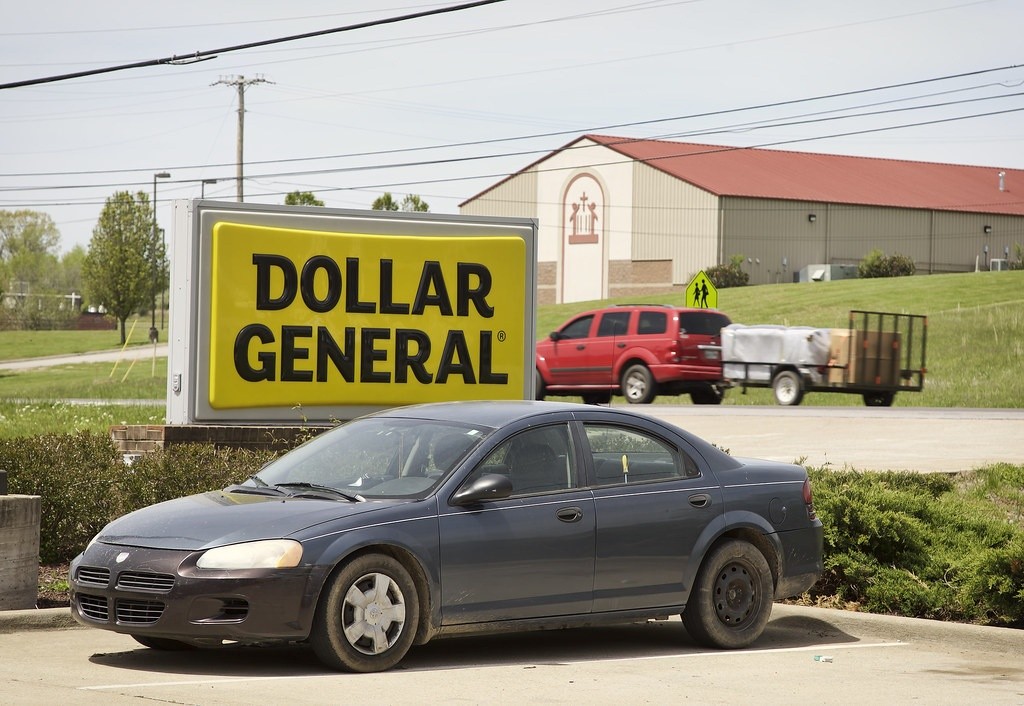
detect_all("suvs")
[535,303,733,404]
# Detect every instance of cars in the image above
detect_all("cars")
[69,400,823,673]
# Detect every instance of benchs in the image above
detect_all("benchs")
[558,455,664,485]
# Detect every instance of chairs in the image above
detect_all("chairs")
[428,433,482,487]
[509,443,561,494]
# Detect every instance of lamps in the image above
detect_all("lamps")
[809,214,816,222]
[984,225,992,233]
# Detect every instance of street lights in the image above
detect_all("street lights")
[201,180,216,199]
[149,172,171,343]
